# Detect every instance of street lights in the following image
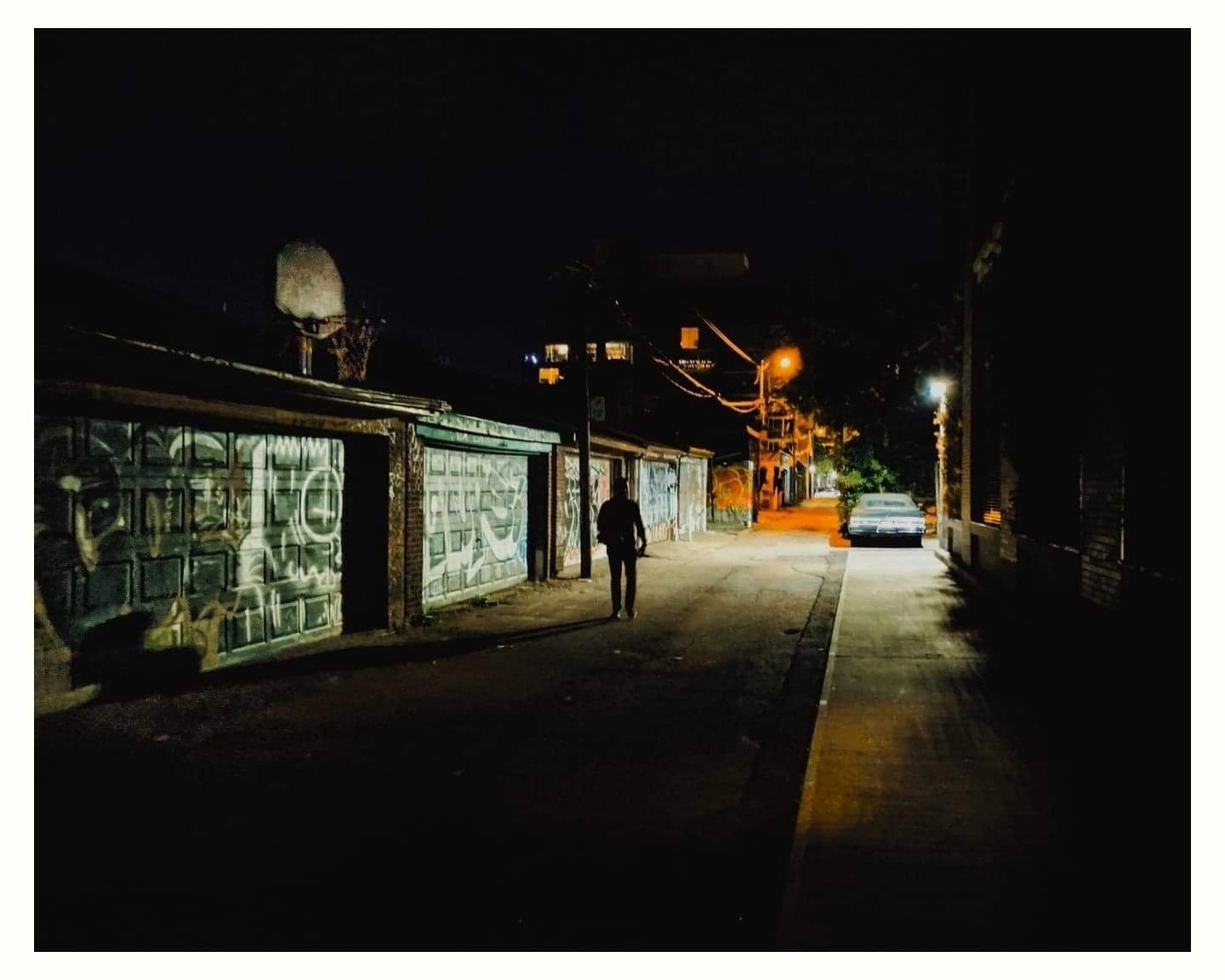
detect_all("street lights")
[759,347,801,419]
[930,381,948,520]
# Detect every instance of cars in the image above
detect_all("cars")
[848,492,926,545]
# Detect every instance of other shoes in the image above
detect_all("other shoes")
[628,608,637,619]
[611,612,620,621]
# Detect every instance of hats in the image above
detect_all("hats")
[613,477,628,490]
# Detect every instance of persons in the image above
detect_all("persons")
[597,478,647,621]
